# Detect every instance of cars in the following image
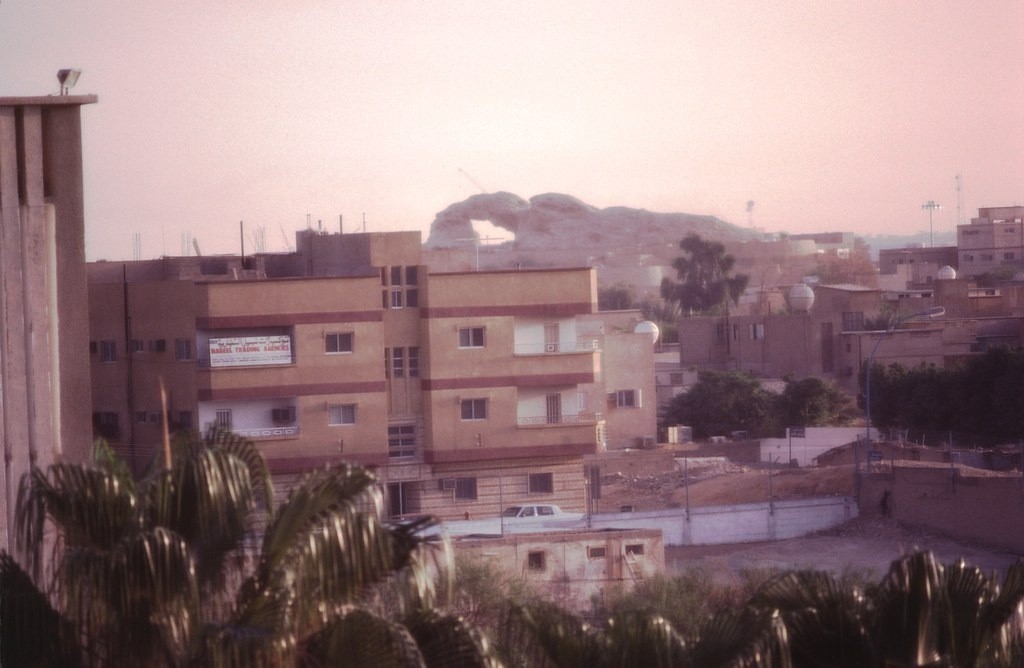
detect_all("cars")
[494,501,588,532]
[383,513,445,544]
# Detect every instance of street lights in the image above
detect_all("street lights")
[921,201,943,249]
[866,304,947,476]
